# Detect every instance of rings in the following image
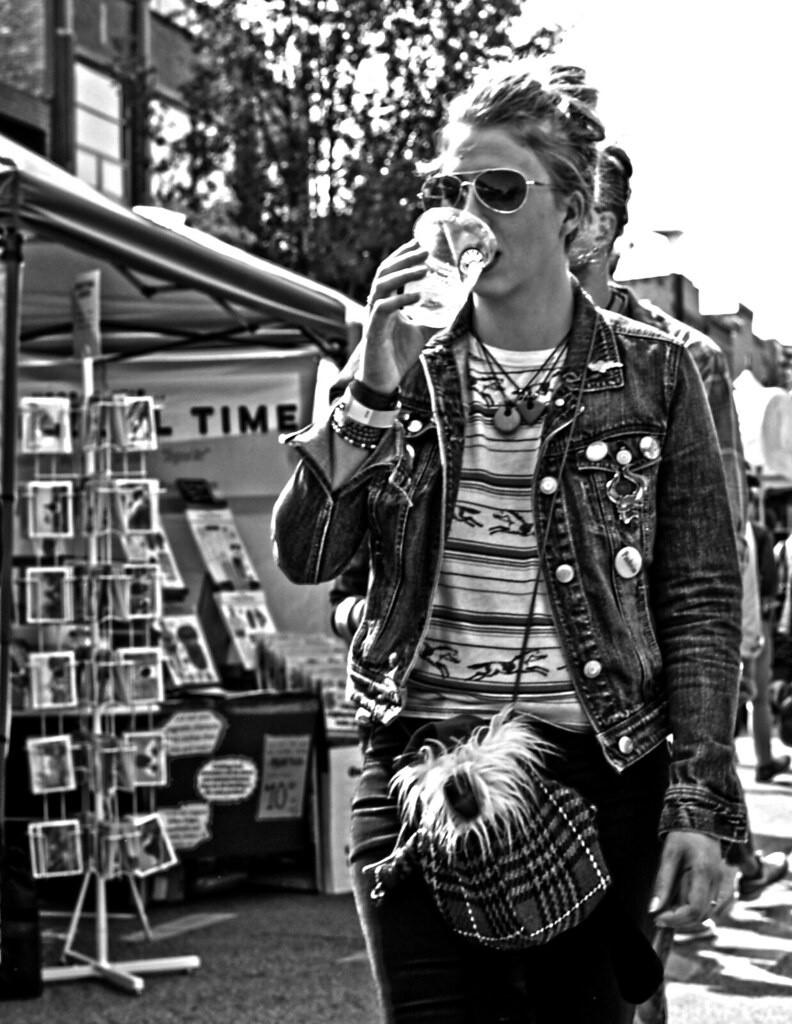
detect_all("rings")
[711,900,718,908]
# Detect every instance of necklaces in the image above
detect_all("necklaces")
[469,324,573,436]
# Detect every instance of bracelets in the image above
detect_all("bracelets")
[330,369,399,452]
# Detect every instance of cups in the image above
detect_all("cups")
[399,205,498,330]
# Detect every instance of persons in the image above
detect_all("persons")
[556,147,749,1024]
[719,469,792,904]
[266,56,745,1024]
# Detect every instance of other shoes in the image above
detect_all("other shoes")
[672,921,715,943]
[739,850,787,899]
[758,755,792,783]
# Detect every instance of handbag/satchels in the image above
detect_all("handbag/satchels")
[384,707,615,951]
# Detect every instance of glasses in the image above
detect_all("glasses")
[415,167,567,218]
